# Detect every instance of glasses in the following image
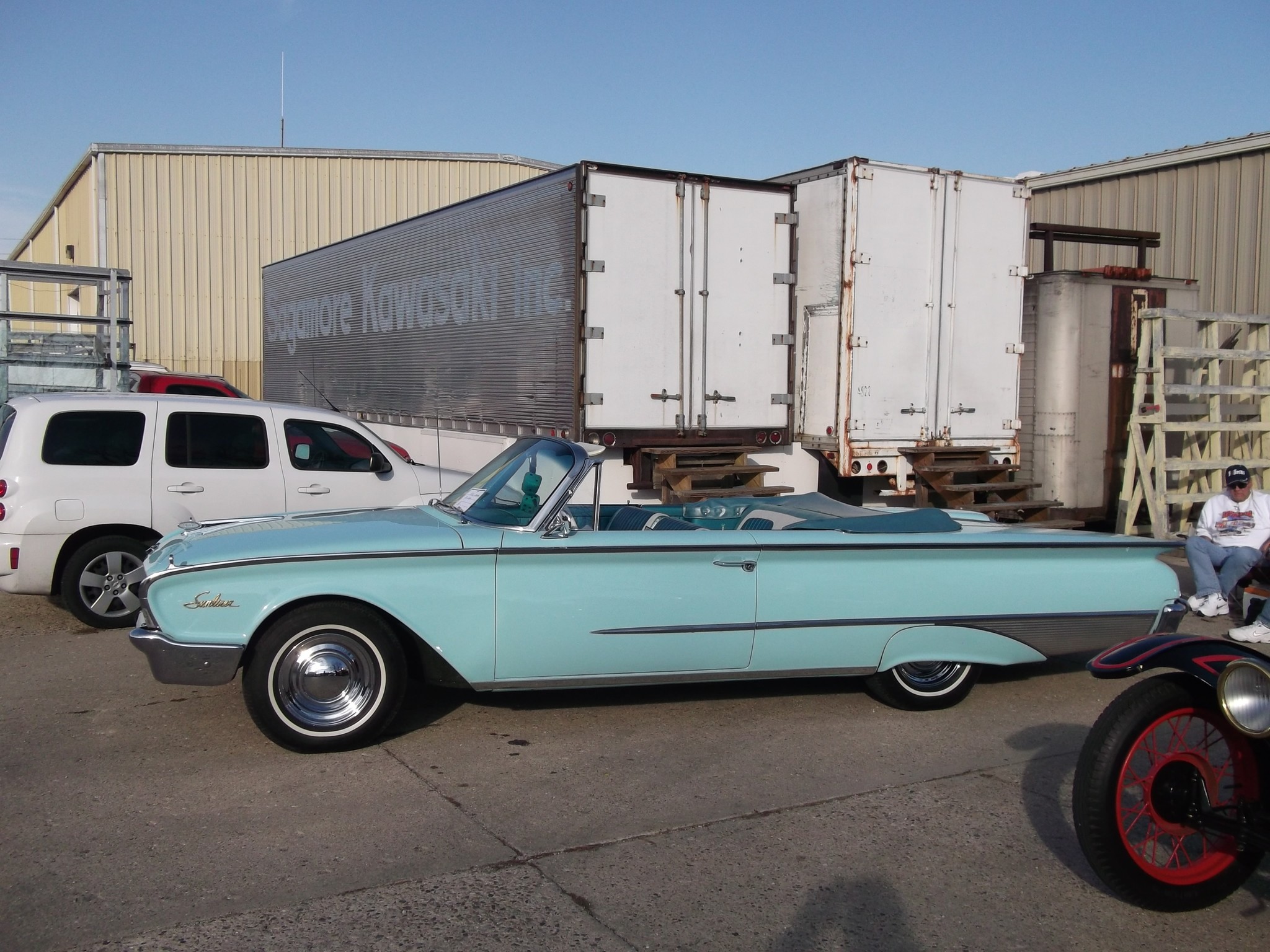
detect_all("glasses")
[1229,480,1250,490]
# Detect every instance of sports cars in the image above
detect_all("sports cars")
[128,435,1190,755]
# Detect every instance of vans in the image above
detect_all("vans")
[0,392,523,631]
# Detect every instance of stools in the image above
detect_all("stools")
[1243,584,1270,619]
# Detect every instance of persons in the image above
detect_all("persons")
[1228,596,1270,643]
[1184,464,1270,616]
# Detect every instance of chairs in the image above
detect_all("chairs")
[1177,534,1270,612]
[605,506,711,531]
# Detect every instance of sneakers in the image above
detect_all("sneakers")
[1196,592,1230,616]
[1187,593,1209,611]
[1228,622,1270,644]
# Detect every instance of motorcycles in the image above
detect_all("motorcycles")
[1072,631,1270,913]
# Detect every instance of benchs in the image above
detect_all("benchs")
[734,504,841,531]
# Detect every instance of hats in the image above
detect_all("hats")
[1226,465,1250,486]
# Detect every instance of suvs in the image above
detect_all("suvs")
[116,360,255,399]
